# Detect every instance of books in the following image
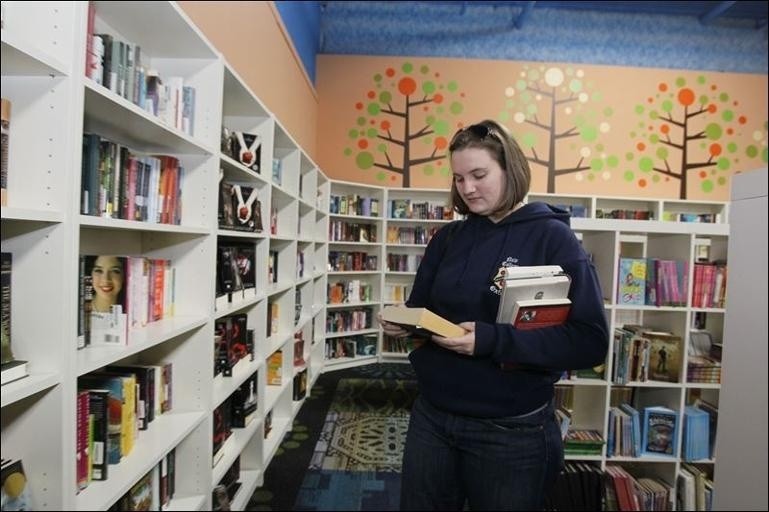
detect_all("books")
[382,198,458,359]
[325,193,379,361]
[554,386,604,454]
[612,324,682,384]
[0,95,30,511]
[382,305,469,342]
[87,2,196,134]
[494,264,573,372]
[76,360,173,494]
[81,133,183,225]
[107,448,176,511]
[265,158,322,437]
[686,355,721,383]
[554,461,713,512]
[596,209,653,220]
[211,123,263,512]
[616,257,688,308]
[78,254,175,348]
[692,261,727,307]
[611,387,719,462]
[663,212,722,224]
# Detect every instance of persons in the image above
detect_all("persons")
[376,118,610,512]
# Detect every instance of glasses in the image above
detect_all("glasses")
[450,125,503,145]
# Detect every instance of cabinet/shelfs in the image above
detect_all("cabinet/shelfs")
[0,0,329,512]
[325,176,466,373]
[710,168,769,511]
